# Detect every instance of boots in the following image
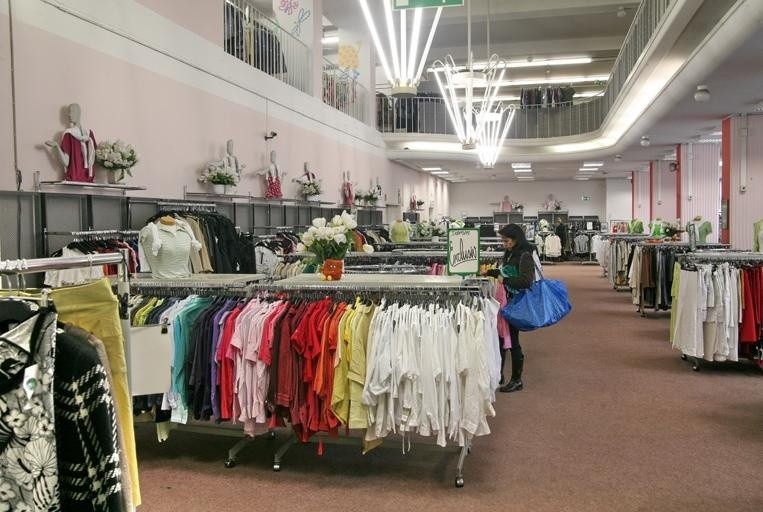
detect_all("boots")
[498,356,525,391]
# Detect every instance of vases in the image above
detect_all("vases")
[213,184,225,194]
[355,199,364,206]
[367,200,372,206]
[107,169,127,184]
[307,195,318,202]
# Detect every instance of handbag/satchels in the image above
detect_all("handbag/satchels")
[500,281,570,332]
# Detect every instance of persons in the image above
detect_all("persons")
[479,224,533,392]
[648,218,670,239]
[45,103,98,182]
[388,218,412,243]
[685,216,713,244]
[628,217,645,235]
[208,140,245,196]
[256,150,287,199]
[291,161,321,196]
[139,215,202,280]
[344,180,353,206]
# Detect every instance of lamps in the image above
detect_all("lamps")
[463,1,516,169]
[359,0,444,99]
[426,0,508,149]
[617,6,625,18]
[641,135,651,146]
[615,155,622,162]
[694,85,710,102]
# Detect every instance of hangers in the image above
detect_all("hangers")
[639,245,761,278]
[377,244,504,254]
[2,244,141,308]
[280,254,506,276]
[110,281,492,322]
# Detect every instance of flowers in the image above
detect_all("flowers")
[198,166,239,186]
[297,210,358,269]
[95,141,138,169]
[301,179,322,195]
[364,187,378,200]
[355,190,364,200]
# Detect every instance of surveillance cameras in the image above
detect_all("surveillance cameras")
[265,129,277,140]
